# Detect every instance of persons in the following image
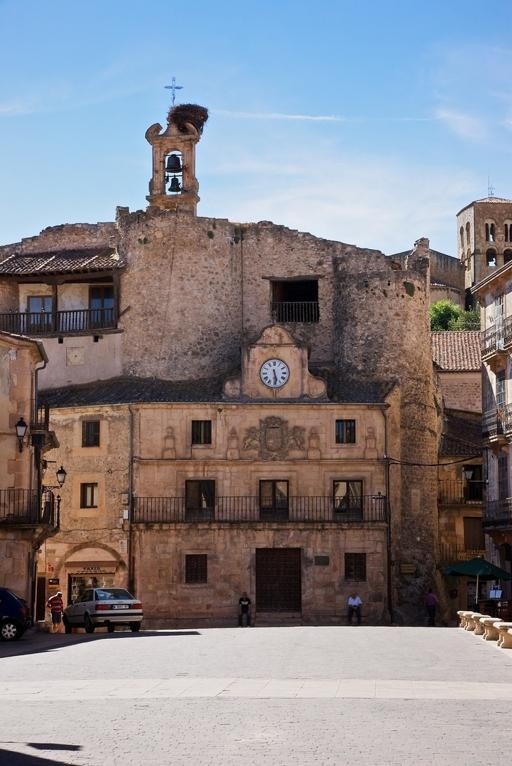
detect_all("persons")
[47,592,64,633]
[424,589,440,627]
[347,591,363,626]
[238,592,251,628]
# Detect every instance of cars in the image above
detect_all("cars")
[1,587,29,641]
[62,587,143,633]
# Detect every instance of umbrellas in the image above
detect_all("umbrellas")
[437,555,511,611]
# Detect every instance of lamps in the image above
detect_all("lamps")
[16,417,29,453]
[42,465,68,493]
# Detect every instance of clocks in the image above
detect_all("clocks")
[259,358,290,390]
